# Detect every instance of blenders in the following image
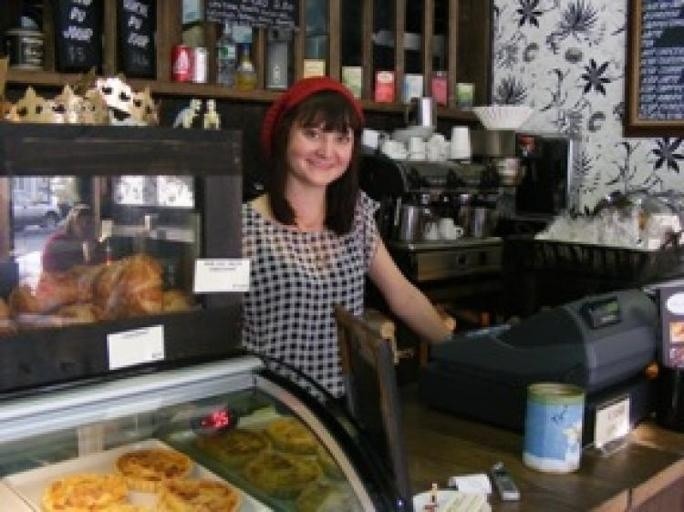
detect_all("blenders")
[468,130,526,193]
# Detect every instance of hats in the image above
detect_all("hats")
[263,77,365,156]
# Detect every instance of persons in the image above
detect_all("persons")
[42,203,109,272]
[240,77,456,406]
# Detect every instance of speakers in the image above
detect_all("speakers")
[515,138,573,217]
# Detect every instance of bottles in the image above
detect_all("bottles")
[457,192,488,239]
[235,45,257,91]
[216,27,237,86]
[394,192,420,244]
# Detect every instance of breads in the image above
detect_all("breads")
[195,415,347,512]
[42,448,242,512]
[0,256,189,331]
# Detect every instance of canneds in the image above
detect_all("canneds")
[171,44,192,82]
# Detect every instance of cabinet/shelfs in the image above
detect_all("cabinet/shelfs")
[0,0,496,511]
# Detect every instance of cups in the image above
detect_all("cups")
[422,222,439,241]
[438,217,463,242]
[450,126,470,159]
